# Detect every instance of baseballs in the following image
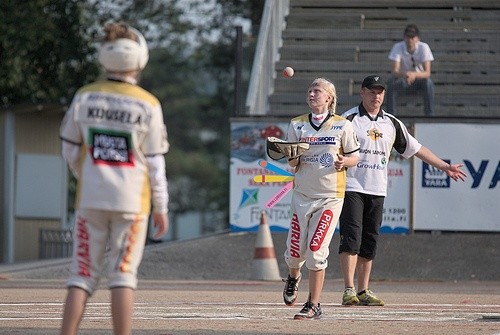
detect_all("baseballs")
[283,66,294,79]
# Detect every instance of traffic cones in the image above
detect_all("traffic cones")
[246,210,284,283]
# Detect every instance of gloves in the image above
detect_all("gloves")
[284,143,304,167]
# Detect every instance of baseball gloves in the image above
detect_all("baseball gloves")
[266,136,311,159]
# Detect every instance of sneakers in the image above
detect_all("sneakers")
[342,287,360,305]
[357,289,384,306]
[294,293,321,319]
[282,273,302,305]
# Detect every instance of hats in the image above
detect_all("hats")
[405,25,419,37]
[362,75,387,91]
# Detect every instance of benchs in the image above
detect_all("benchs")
[265,0,500,117]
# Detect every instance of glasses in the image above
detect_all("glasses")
[412,57,415,68]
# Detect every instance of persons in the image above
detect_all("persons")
[386,24,435,118]
[281,77,361,321]
[58,23,169,335]
[339,74,468,307]
